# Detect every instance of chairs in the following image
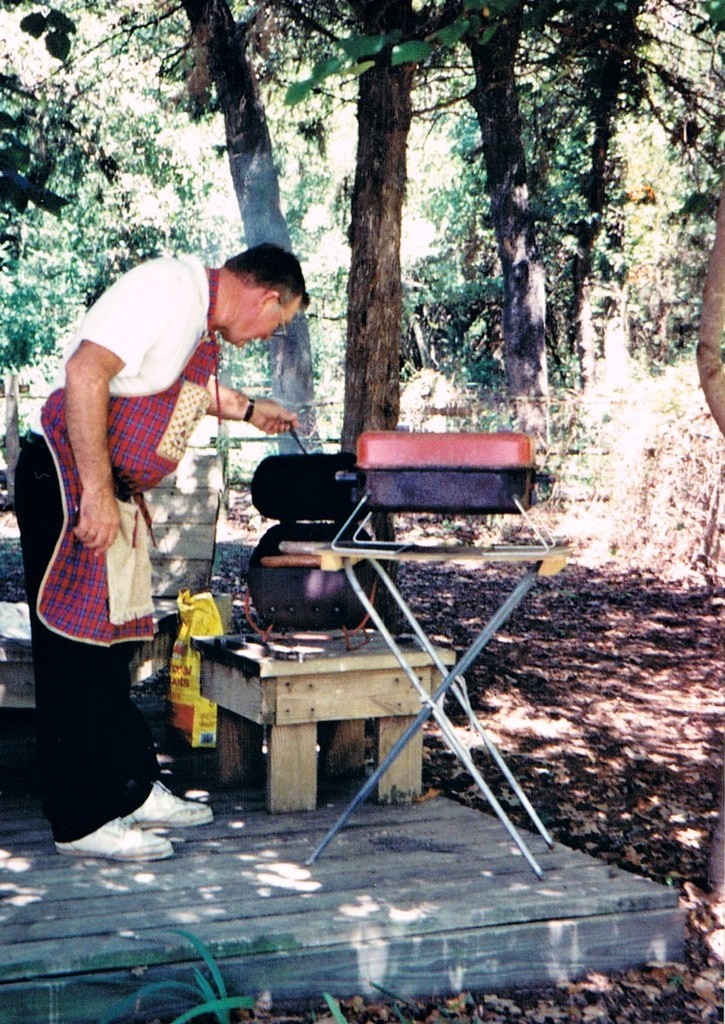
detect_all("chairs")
[0,454,228,708]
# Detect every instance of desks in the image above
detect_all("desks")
[190,628,455,813]
[306,541,575,882]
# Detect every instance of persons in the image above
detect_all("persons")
[14,244,312,864]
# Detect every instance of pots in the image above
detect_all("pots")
[252,454,368,521]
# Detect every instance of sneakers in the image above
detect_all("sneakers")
[125,780,213,831]
[53,816,173,862]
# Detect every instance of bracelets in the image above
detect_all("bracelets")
[244,397,257,423]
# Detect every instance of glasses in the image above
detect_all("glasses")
[271,292,287,337]
